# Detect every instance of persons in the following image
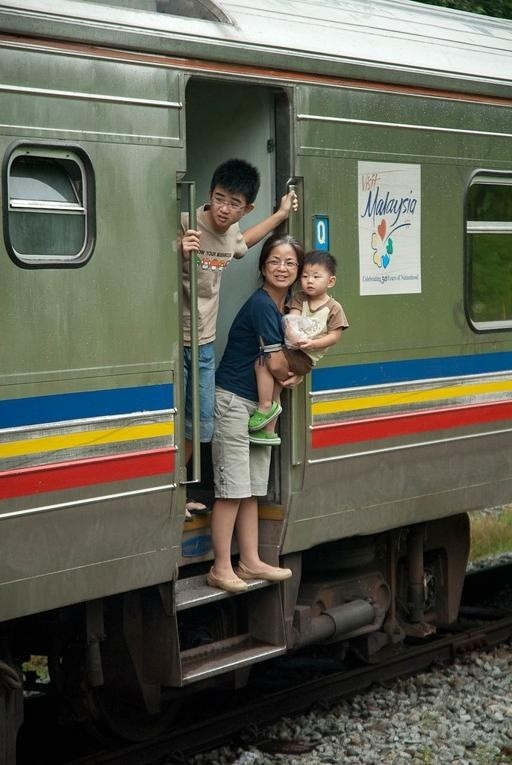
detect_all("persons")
[180,158,299,522]
[207,233,304,592]
[248,250,349,446]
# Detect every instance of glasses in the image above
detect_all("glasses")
[213,197,248,211]
[267,260,300,270]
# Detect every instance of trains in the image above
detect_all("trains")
[0,0,512,765]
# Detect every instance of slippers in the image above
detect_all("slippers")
[185,498,210,521]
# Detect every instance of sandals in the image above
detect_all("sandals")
[248,401,283,446]
[206,560,292,592]
